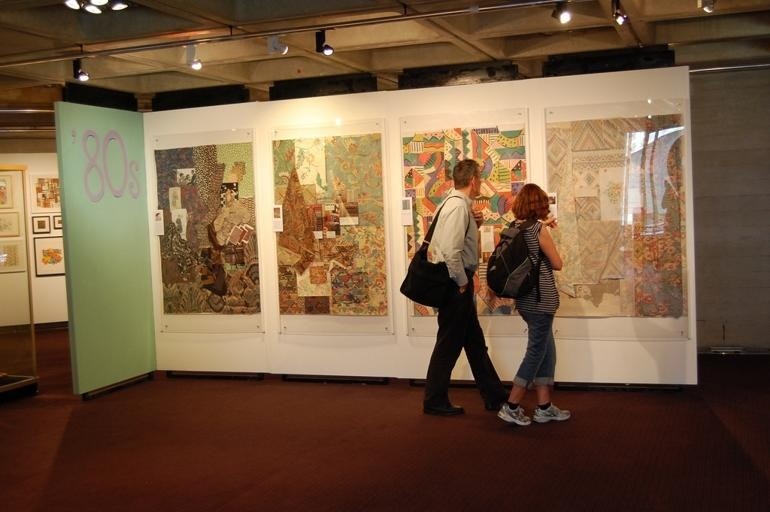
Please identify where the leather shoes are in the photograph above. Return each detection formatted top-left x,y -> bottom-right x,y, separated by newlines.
421,400 -> 464,416
483,387 -> 510,410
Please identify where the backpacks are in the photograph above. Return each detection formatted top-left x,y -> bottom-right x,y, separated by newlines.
485,217 -> 545,299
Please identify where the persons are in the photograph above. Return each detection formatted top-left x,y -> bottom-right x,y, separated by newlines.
423,159 -> 510,416
497,184 -> 571,426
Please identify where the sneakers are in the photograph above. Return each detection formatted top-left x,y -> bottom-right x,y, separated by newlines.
532,402 -> 571,423
496,402 -> 532,426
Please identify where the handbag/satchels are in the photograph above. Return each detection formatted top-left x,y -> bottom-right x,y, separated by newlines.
399,195 -> 471,310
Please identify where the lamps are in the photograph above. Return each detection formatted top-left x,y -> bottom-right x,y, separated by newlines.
315,30 -> 333,56
552,3 -> 571,23
73,58 -> 90,82
697,1 -> 716,13
64,1 -> 129,15
610,0 -> 628,25
186,44 -> 202,69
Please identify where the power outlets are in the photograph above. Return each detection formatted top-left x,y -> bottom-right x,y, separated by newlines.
271,37 -> 288,56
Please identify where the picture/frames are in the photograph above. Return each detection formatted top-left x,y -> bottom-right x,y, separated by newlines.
1,174 -> 25,274
29,173 -> 66,276
